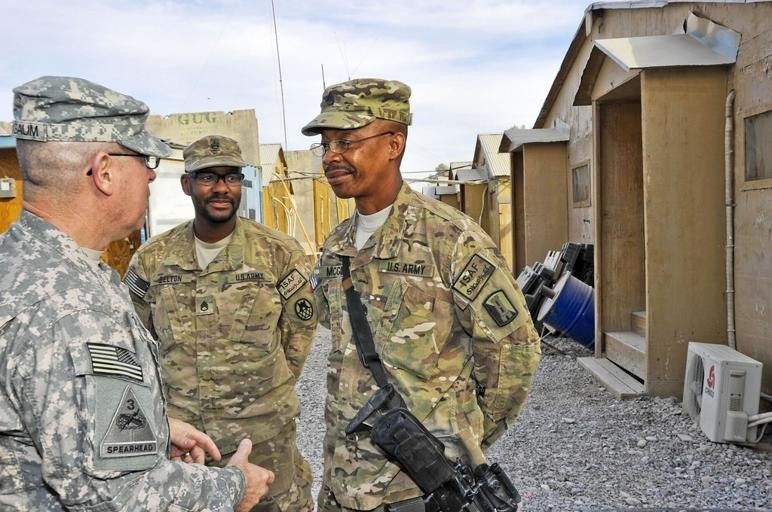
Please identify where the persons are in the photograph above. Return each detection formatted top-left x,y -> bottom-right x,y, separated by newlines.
121,136 -> 321,510
301,80 -> 541,512
0,76 -> 275,511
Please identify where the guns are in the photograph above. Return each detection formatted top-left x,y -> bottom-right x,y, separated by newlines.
340,386 -> 522,512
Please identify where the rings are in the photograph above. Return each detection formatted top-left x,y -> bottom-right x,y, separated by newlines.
181,452 -> 191,460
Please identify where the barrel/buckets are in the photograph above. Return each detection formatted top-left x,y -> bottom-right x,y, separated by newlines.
537,271 -> 595,352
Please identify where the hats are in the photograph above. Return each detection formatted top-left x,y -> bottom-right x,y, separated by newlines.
11,76 -> 174,159
301,78 -> 413,136
182,135 -> 247,173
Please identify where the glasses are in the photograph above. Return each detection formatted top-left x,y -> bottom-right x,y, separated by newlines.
86,153 -> 160,176
308,133 -> 392,157
191,172 -> 244,187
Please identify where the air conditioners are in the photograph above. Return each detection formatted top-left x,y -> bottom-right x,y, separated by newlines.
681,341 -> 772,447
